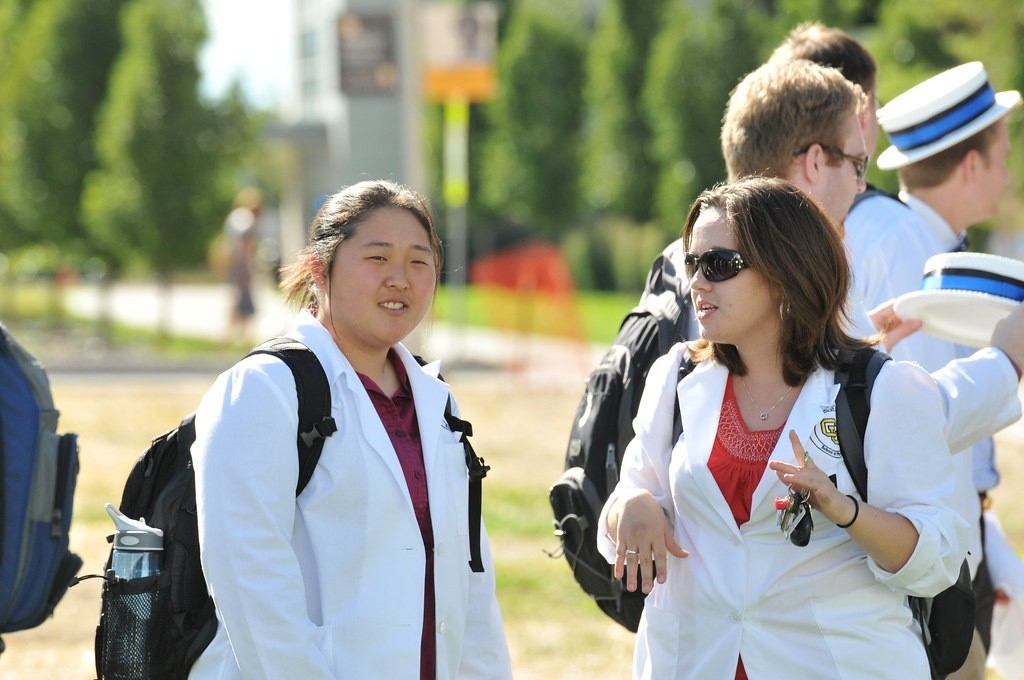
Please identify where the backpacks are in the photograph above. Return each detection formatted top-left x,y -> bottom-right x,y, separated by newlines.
96,411 -> 217,680
0,324 -> 85,640
907,551 -> 976,679
550,241 -> 692,633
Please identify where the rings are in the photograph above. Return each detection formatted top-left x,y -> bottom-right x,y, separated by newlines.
625,548 -> 638,555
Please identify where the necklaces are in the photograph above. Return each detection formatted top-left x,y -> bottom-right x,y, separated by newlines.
741,372 -> 795,420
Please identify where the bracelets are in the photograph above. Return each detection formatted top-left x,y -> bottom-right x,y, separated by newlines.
835,494 -> 859,528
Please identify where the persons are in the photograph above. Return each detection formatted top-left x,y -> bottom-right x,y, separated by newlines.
181,180 -> 515,680
873,61 -> 1024,674
596,175 -> 968,680
771,21 -> 924,341
637,58 -> 1023,456
224,184 -> 262,344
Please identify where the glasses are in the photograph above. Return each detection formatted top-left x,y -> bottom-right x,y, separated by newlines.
789,141 -> 873,180
683,247 -> 750,282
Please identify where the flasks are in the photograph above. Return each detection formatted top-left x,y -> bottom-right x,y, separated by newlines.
103,504 -> 163,680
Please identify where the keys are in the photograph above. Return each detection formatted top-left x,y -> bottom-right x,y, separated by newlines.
774,450 -> 815,548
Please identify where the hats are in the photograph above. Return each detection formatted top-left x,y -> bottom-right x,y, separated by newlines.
893,250 -> 1024,347
870,62 -> 1024,171
